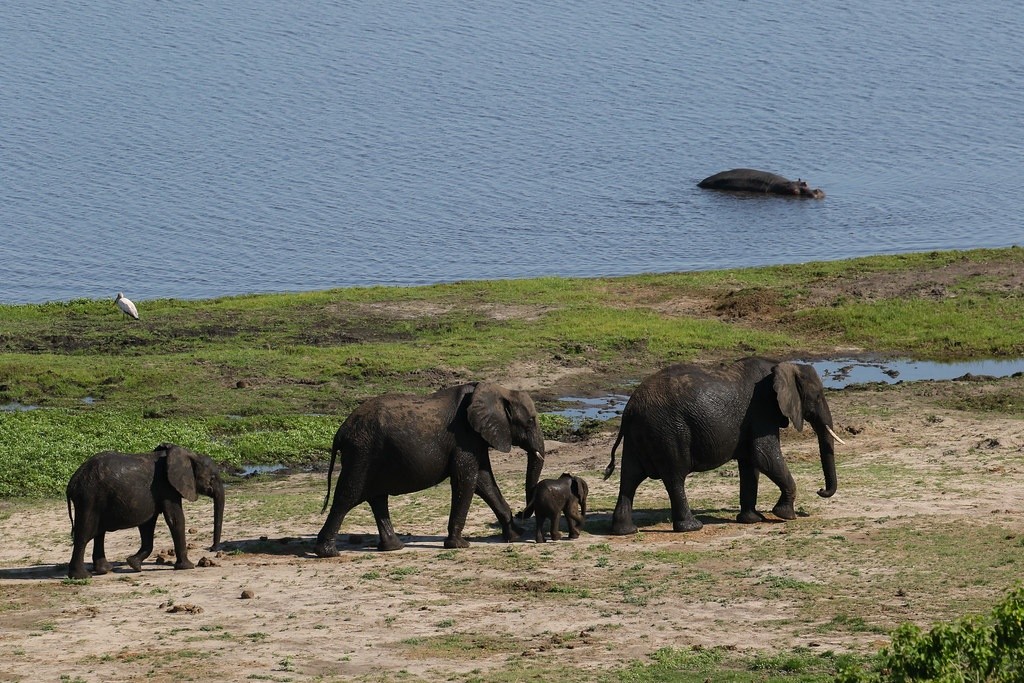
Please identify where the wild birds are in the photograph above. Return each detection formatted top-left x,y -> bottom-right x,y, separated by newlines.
113,292 -> 139,321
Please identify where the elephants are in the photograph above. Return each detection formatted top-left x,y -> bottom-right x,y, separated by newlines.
315,355 -> 846,559
66,441 -> 225,580
